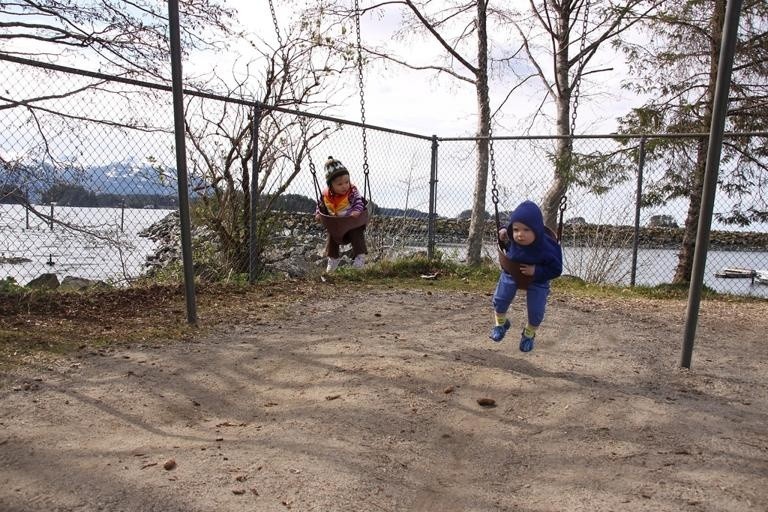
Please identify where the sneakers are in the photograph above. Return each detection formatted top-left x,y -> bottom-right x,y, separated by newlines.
490,319 -> 511,342
352,254 -> 365,269
519,327 -> 535,352
326,257 -> 340,273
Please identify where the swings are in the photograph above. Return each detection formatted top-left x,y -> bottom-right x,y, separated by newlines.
475,0 -> 591,289
269,0 -> 374,244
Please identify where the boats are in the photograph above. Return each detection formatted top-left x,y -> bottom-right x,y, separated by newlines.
712,265 -> 767,285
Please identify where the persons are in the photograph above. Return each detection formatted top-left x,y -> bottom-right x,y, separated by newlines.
491,200 -> 563,352
316,156 -> 368,273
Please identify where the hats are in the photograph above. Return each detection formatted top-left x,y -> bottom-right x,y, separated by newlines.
324,156 -> 349,186
